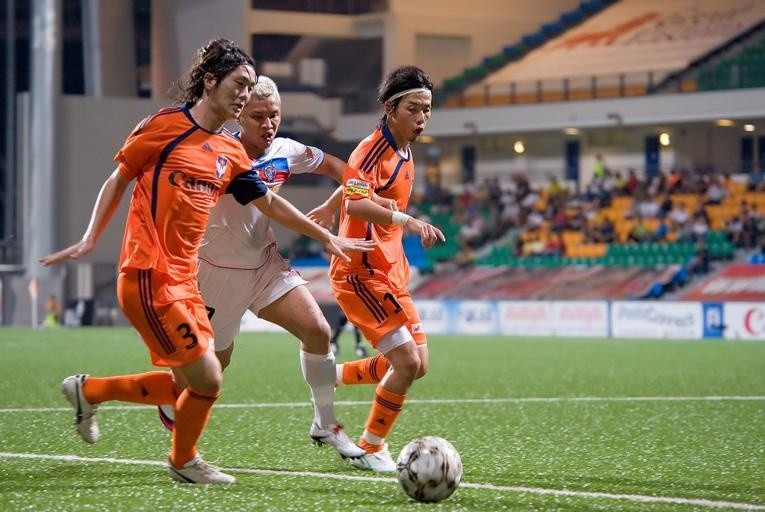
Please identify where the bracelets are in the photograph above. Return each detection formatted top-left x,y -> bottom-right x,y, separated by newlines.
389,210 -> 413,226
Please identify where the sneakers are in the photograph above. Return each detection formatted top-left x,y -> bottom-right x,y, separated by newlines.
167,454 -> 235,483
60,374 -> 101,443
309,421 -> 366,459
352,443 -> 396,473
157,404 -> 176,429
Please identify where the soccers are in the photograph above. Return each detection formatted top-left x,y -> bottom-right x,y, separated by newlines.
397,437 -> 462,503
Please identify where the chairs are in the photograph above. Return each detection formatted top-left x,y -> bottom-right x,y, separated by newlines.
277,202 -> 732,265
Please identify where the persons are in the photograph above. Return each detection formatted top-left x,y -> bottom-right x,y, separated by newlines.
423,152 -> 765,274
159,74 -> 400,461
45,291 -> 61,326
38,39 -> 380,486
304,65 -> 447,473
